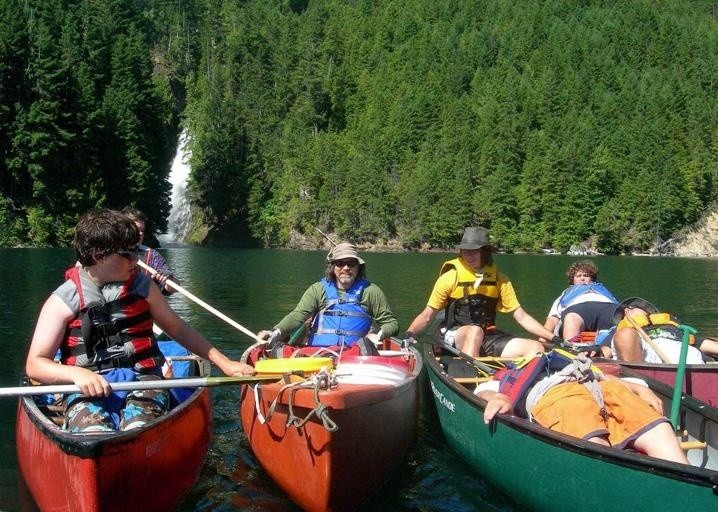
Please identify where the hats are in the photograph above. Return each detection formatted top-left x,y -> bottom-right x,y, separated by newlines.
326,243 -> 366,264
452,227 -> 499,252
612,297 -> 660,325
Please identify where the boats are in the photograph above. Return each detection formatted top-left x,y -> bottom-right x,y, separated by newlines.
15,353 -> 213,512
239,335 -> 423,512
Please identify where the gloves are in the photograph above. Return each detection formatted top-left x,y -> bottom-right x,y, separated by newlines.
257,329 -> 279,345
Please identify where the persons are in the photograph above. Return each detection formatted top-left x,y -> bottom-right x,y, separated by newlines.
121,209 -> 180,341
256,244 -> 400,350
475,349 -> 692,467
577,298 -> 718,364
24,208 -> 257,436
540,261 -> 621,343
399,228 -> 571,371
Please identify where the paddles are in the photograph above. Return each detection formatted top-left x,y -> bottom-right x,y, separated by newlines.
1,375 -> 283,395
420,330 -> 498,374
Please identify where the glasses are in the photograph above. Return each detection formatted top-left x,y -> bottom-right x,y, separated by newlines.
335,260 -> 358,268
101,242 -> 140,261
